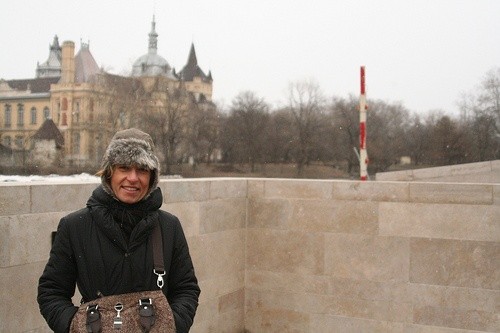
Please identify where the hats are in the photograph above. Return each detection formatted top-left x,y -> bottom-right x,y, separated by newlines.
100,128 -> 159,202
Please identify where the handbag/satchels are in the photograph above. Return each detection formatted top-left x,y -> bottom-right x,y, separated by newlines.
69,290 -> 176,333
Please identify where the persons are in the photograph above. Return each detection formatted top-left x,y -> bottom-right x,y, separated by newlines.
37,128 -> 201,333
